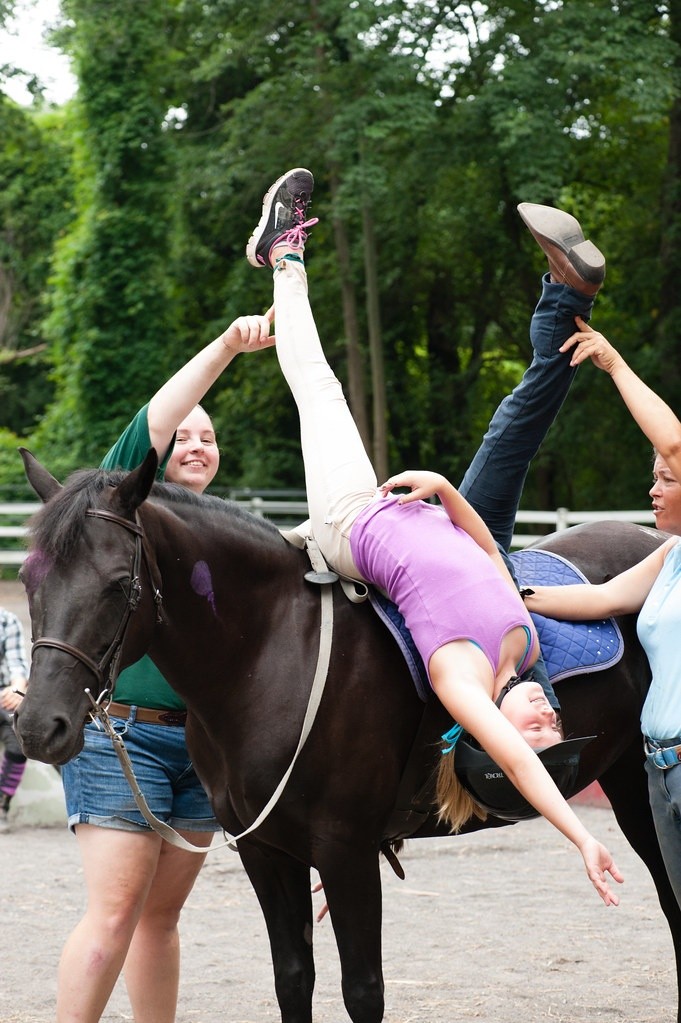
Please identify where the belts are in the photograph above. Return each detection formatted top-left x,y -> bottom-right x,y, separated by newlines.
99,700 -> 187,728
644,742 -> 681,770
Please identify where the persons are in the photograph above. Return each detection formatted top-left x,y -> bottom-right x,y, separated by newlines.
0,606 -> 32,833
516,316 -> 681,913
52,299 -> 278,1023
455,201 -> 609,736
244,167 -> 624,908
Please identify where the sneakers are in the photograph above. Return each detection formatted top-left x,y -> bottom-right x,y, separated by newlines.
245,168 -> 315,267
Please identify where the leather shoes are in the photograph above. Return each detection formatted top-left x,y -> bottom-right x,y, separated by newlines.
516,202 -> 606,297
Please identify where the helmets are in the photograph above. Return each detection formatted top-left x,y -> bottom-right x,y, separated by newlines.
454,735 -> 616,822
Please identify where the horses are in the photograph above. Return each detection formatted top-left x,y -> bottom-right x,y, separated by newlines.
13,445 -> 680,1023
